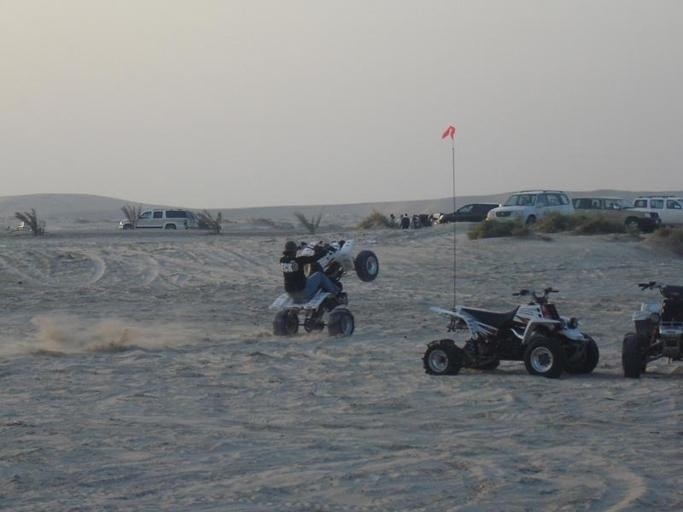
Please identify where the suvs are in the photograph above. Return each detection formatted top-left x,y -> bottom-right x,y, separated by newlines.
117,207 -> 193,232
630,195 -> 683,227
571,196 -> 663,234
438,203 -> 501,226
485,189 -> 577,228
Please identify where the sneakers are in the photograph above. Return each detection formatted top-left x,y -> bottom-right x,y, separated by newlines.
335,282 -> 342,297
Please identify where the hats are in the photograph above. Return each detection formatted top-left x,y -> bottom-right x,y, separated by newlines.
283,241 -> 297,254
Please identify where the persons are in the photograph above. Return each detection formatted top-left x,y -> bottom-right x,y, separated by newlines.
388,213 -> 443,230
279,241 -> 345,304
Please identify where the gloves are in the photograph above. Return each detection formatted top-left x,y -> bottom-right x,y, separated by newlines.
316,247 -> 327,256
